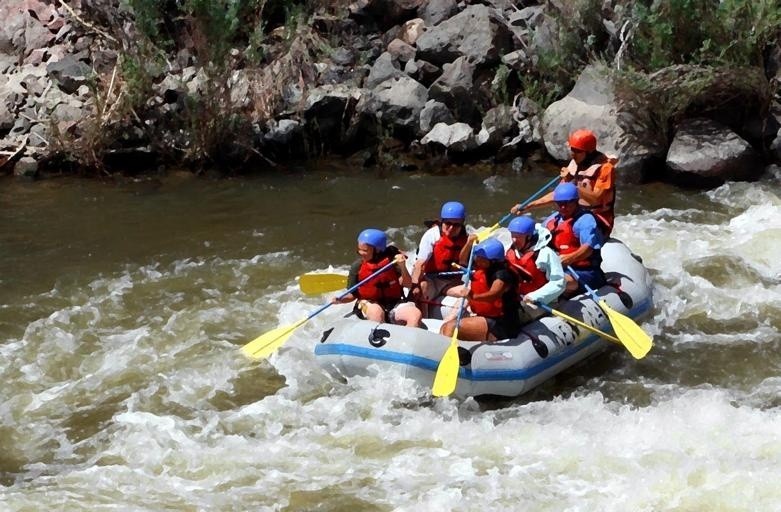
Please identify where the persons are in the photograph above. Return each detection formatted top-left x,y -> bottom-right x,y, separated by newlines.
540,182 -> 602,301
329,228 -> 423,328
510,128 -> 616,245
439,237 -> 521,343
408,201 -> 469,318
504,216 -> 566,327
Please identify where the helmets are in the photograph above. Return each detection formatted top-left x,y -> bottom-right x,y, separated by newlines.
508,216 -> 534,234
473,239 -> 504,260
554,183 -> 578,201
569,130 -> 596,152
358,229 -> 386,253
441,201 -> 464,219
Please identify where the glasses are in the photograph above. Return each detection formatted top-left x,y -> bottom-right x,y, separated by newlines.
571,147 -> 582,154
442,220 -> 462,227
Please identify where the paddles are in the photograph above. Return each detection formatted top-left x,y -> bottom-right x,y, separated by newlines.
432,234 -> 476,397
238,254 -> 398,359
452,263 -> 621,345
299,273 -> 476,295
554,250 -> 652,359
473,175 -> 561,243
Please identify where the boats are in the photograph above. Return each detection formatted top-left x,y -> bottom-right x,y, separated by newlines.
310,237 -> 653,402
